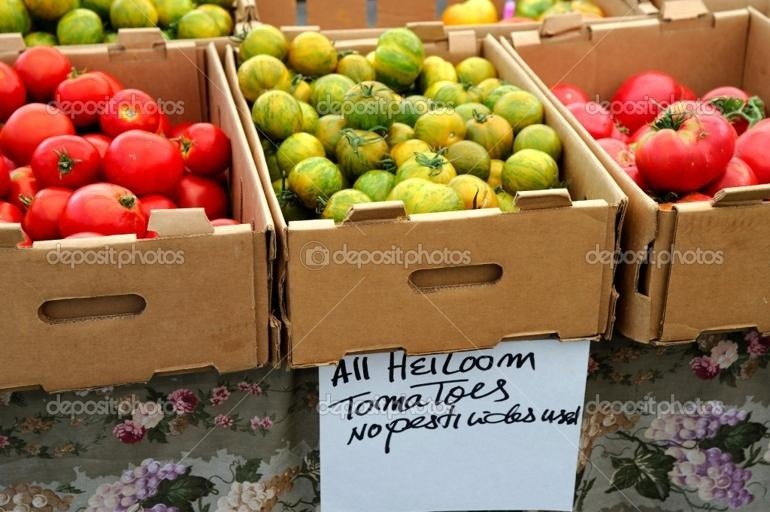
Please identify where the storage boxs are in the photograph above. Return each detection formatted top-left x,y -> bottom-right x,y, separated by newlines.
503,2 -> 770,347
0,29 -> 277,399
227,30 -> 634,373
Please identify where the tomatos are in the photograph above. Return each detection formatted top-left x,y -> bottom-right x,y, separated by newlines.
0,0 -> 770,247
